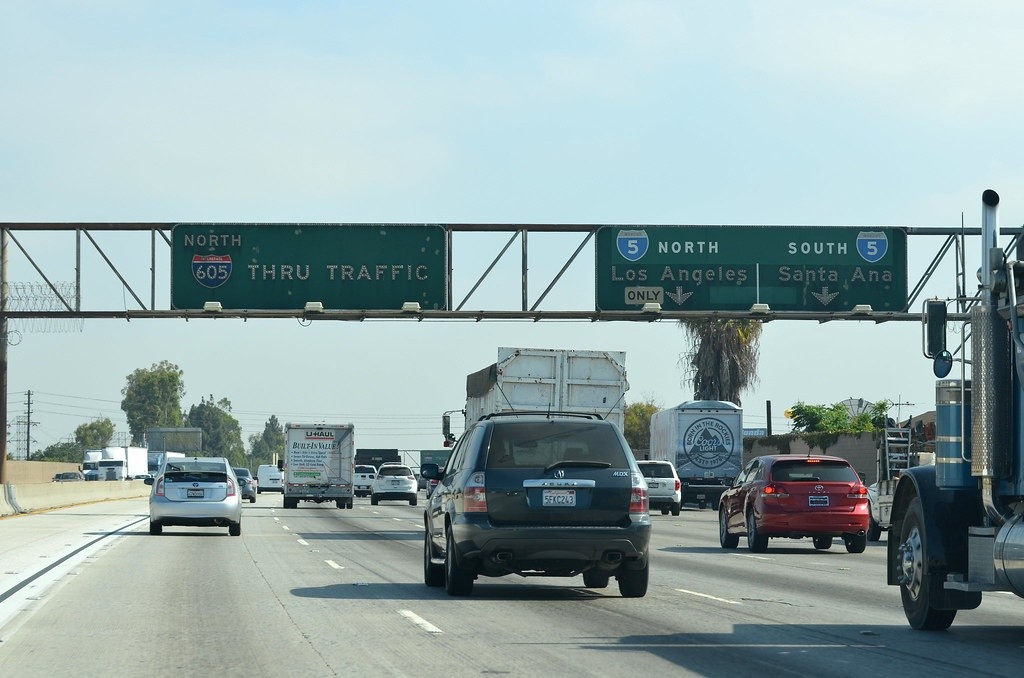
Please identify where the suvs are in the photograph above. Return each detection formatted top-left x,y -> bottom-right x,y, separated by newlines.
636,459 -> 682,516
420,410 -> 652,597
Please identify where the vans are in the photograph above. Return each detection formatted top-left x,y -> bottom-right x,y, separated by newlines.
256,464 -> 284,496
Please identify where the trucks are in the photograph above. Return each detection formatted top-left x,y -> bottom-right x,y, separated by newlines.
277,422 -> 355,509
645,401 -> 744,511
887,189 -> 1024,631
868,427 -> 921,541
443,346 -> 630,446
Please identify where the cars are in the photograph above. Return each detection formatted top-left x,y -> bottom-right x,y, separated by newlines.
352,464 -> 377,497
369,464 -> 418,506
52,473 -> 86,482
719,454 -> 870,554
233,466 -> 259,503
144,456 -> 246,536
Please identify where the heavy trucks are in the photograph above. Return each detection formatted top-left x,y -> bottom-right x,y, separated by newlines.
97,447 -> 148,480
147,451 -> 185,478
78,450 -> 102,481
